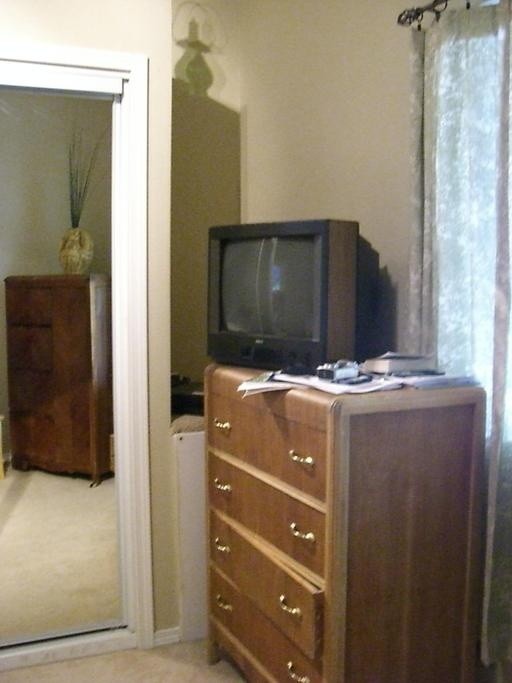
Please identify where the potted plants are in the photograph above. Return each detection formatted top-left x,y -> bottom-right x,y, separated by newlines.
60,120 -> 111,274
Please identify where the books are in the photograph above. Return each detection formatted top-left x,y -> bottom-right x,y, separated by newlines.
363,351 -> 436,375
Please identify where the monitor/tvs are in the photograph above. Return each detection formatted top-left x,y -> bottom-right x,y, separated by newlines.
205,218 -> 391,377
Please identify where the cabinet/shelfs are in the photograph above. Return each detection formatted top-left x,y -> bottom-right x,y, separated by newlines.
203,368 -> 486,683
7,274 -> 113,485
